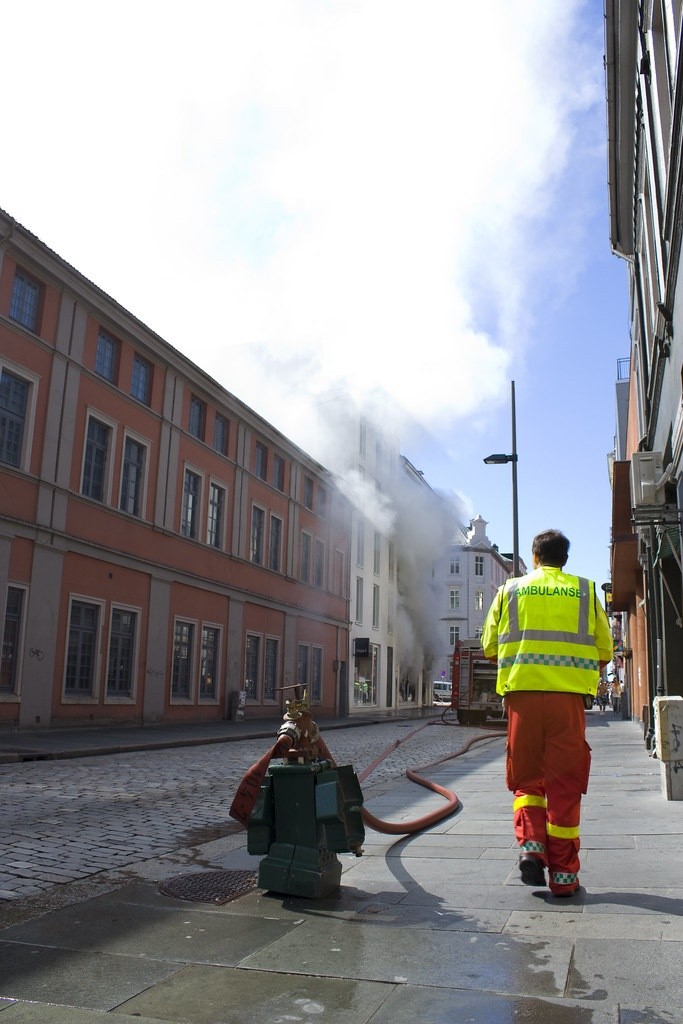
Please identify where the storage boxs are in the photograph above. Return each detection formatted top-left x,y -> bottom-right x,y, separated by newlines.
660,760 -> 683,802
652,695 -> 683,762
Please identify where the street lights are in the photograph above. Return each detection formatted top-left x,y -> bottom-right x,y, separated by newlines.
482,381 -> 519,577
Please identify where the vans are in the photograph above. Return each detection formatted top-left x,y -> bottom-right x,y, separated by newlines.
432,683 -> 453,701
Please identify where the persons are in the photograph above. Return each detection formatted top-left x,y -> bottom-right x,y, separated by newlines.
481,530 -> 624,895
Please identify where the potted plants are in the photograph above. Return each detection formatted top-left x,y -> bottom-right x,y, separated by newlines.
621,646 -> 632,659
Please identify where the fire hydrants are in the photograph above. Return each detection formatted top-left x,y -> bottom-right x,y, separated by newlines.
247,692 -> 365,898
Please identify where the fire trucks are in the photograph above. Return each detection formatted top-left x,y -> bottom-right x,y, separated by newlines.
452,638 -> 503,726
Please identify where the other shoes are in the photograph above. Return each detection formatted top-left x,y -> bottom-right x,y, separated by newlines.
600,711 -> 604,716
518,855 -> 547,887
